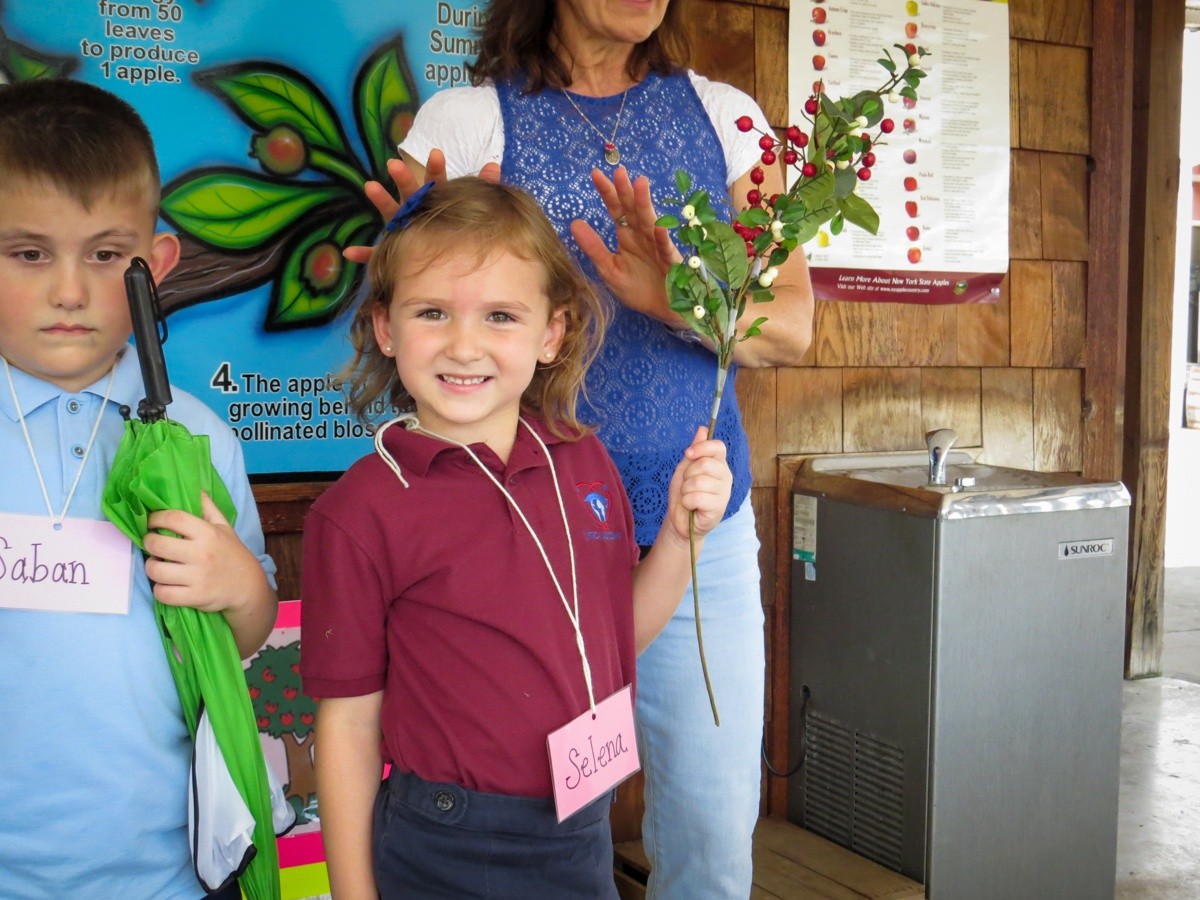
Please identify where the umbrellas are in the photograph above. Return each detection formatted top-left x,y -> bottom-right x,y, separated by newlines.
105,253 -> 279,900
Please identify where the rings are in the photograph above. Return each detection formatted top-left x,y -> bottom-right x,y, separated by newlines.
615,214 -> 629,227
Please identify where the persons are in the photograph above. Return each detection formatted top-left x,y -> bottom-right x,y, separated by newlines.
300,176 -> 735,900
0,77 -> 278,900
343,0 -> 815,900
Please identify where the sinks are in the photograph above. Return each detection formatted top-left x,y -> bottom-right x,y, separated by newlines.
843,464 -> 1059,495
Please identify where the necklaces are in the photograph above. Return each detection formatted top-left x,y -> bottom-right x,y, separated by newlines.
549,68 -> 631,165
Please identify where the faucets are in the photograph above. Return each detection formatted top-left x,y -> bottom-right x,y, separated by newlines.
924,427 -> 958,486
